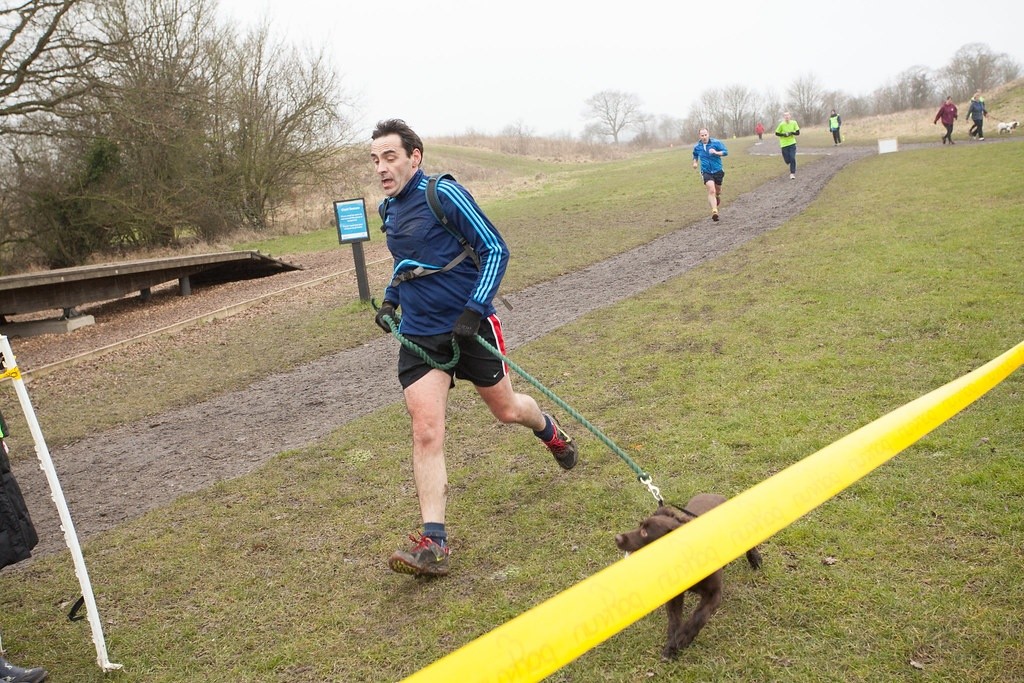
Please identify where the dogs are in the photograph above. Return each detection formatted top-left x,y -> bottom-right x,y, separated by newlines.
998,121 -> 1019,135
614,493 -> 763,660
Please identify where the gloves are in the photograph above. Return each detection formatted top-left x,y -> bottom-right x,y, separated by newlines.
451,310 -> 480,341
375,302 -> 398,333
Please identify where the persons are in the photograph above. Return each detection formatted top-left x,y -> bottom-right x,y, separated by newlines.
966,89 -> 988,141
693,129 -> 728,222
756,123 -> 764,139
0,407 -> 48,683
934,97 -> 958,144
829,110 -> 842,144
776,112 -> 801,179
374,119 -> 578,578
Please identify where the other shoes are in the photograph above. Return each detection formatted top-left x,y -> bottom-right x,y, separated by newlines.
715,192 -> 721,205
790,173 -> 795,180
943,137 -> 946,144
971,134 -> 976,140
979,137 -> 985,141
0,657 -> 47,683
712,211 -> 719,221
949,139 -> 955,144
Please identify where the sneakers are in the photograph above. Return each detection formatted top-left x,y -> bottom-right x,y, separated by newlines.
539,413 -> 578,470
388,530 -> 450,575
